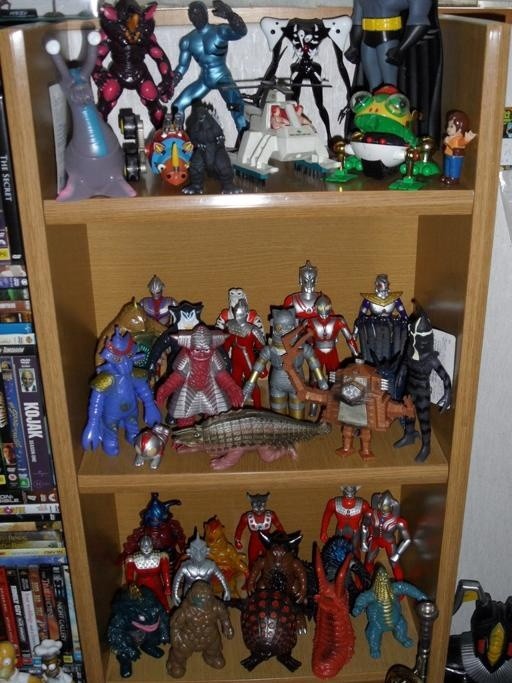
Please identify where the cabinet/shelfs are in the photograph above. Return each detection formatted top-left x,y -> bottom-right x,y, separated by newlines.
0,7 -> 511,683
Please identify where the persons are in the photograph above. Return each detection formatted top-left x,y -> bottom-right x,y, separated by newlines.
33,639 -> 76,683
0,641 -> 31,683
20,371 -> 36,392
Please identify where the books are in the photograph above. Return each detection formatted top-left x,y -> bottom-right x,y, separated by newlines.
0,513 -> 83,683
1,88 -> 37,346
3,346 -> 61,515
